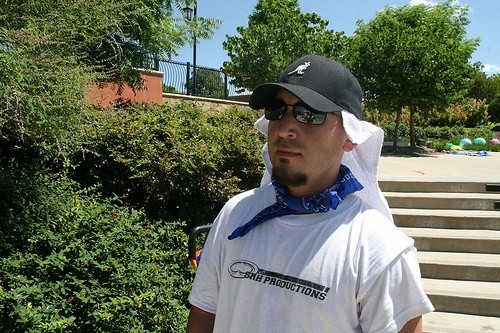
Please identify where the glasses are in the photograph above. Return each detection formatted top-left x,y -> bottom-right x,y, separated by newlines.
264,98 -> 342,126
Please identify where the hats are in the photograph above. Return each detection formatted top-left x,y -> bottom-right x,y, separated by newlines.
247,55 -> 363,122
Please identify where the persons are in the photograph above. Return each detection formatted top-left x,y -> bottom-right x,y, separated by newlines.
186,54 -> 436,333
